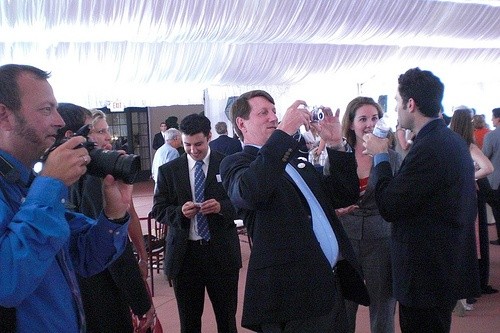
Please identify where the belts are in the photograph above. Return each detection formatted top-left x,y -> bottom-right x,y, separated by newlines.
189,240 -> 209,245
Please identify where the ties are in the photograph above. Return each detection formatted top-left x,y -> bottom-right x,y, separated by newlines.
193,160 -> 211,242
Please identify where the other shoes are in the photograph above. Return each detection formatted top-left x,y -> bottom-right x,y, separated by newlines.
485,285 -> 499,295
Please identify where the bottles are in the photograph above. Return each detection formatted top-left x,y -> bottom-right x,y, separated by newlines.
372,112 -> 392,139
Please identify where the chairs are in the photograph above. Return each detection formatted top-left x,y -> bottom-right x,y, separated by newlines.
131,210 -> 167,289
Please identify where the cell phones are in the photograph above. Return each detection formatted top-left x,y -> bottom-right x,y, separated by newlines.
193,203 -> 202,207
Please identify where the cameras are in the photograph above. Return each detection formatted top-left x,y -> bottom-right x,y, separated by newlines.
53,124 -> 141,184
303,105 -> 324,122
